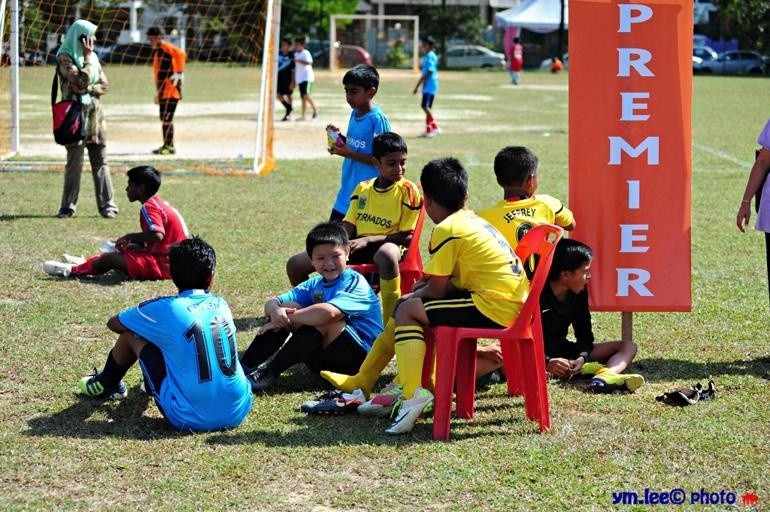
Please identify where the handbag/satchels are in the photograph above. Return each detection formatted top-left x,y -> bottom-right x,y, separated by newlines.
154,90 -> 160,104
53,100 -> 85,145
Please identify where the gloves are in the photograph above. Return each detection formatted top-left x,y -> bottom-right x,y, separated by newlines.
169,72 -> 184,87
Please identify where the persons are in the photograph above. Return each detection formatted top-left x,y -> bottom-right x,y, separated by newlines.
56,20 -> 119,218
326,64 -> 392,223
507,37 -> 525,84
733,110 -> 769,305
235,131 -> 650,435
145,27 -> 188,155
412,36 -> 443,137
1,32 -> 63,67
43,165 -> 192,281
291,37 -> 318,121
277,37 -> 296,121
548,54 -> 564,73
78,234 -> 254,432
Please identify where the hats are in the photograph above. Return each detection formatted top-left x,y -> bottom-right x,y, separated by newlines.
147,27 -> 165,35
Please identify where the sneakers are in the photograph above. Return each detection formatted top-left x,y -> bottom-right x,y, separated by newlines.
423,126 -> 441,138
587,367 -> 644,394
100,211 -> 115,218
282,108 -> 319,122
152,144 -> 175,154
580,361 -> 603,375
57,209 -> 71,218
140,372 -> 146,393
79,367 -> 128,402
301,383 -> 434,433
43,253 -> 86,277
240,362 -> 280,393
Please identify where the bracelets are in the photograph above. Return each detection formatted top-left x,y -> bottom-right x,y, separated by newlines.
741,197 -> 752,205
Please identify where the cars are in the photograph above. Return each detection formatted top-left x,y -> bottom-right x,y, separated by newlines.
311,45 -> 372,68
693,47 -> 717,60
701,50 -> 766,76
403,46 -> 508,72
693,55 -> 702,73
539,53 -> 569,71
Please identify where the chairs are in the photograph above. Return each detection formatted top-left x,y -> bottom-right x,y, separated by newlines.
348,182 -> 424,294
423,223 -> 565,440
